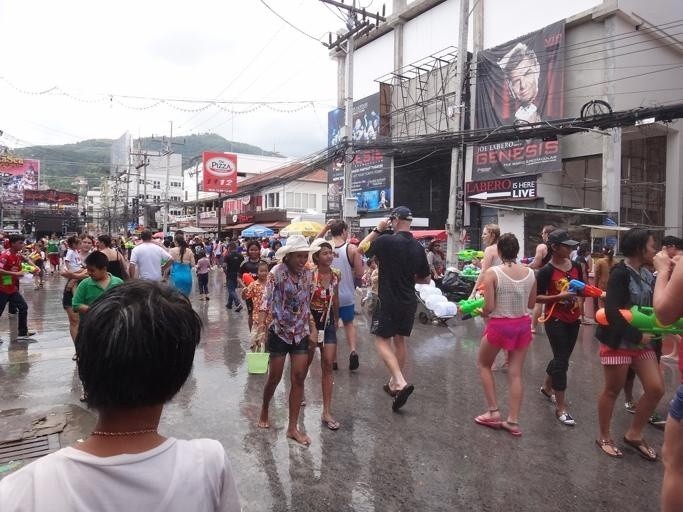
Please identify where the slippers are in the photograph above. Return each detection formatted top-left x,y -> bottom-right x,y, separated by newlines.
624,434 -> 658,461
323,415 -> 339,430
384,383 -> 414,410
555,408 -> 575,426
596,437 -> 623,458
539,386 -> 558,404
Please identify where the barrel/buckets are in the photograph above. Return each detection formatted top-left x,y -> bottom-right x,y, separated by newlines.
414,279 -> 457,317
246,343 -> 271,374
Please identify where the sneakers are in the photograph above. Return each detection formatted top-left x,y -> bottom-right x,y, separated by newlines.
624,400 -> 637,413
19,332 -> 36,337
649,410 -> 666,425
226,304 -> 242,312
349,351 -> 359,370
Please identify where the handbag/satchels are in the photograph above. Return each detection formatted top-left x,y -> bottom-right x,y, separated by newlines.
316,330 -> 325,347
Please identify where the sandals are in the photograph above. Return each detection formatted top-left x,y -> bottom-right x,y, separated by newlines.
474,409 -> 522,436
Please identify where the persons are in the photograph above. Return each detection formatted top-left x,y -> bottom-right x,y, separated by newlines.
377,190 -> 390,210
496,42 -> 542,129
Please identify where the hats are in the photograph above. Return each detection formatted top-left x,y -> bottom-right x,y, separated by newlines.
550,229 -> 580,246
309,238 -> 335,265
275,235 -> 320,260
386,206 -> 413,221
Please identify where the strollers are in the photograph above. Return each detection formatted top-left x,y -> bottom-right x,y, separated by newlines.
435,266 -> 474,301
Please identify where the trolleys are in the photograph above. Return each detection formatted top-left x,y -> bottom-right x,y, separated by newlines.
415,288 -> 456,327
